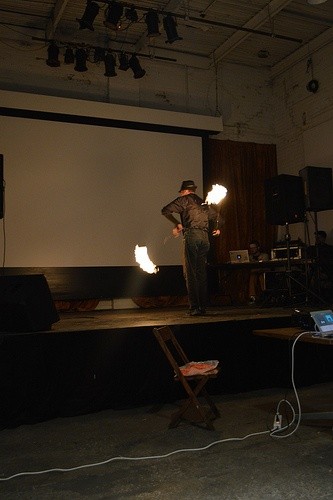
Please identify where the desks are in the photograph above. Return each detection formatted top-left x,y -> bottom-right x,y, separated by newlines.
254,326 -> 333,422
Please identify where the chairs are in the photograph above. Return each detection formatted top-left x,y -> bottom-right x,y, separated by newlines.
153,324 -> 225,431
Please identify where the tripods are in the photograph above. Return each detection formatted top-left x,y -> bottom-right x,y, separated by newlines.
257,223 -> 331,309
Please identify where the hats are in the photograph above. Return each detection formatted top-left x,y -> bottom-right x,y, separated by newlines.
314,231 -> 327,237
178,181 -> 196,193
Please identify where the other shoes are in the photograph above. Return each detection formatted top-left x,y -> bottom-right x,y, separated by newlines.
199,306 -> 206,315
248,296 -> 256,305
185,306 -> 201,316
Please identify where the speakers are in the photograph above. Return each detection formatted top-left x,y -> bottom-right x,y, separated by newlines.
264,174 -> 306,226
0,274 -> 58,332
305,166 -> 333,212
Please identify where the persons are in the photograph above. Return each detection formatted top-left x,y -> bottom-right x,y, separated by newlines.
314,231 -> 327,248
161,180 -> 224,317
248,242 -> 269,304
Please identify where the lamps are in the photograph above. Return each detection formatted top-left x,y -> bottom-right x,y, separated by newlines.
75,1 -> 100,31
103,3 -> 124,30
74,47 -> 88,72
118,53 -> 130,72
94,48 -> 105,64
127,7 -> 138,20
47,42 -> 61,67
105,51 -> 117,77
129,54 -> 145,79
163,14 -> 183,44
65,45 -> 75,64
145,8 -> 161,38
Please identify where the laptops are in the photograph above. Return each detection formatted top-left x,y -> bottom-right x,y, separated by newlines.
229,250 -> 251,264
310,310 -> 333,337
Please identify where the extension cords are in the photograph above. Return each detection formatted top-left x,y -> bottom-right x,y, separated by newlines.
274,414 -> 283,429
